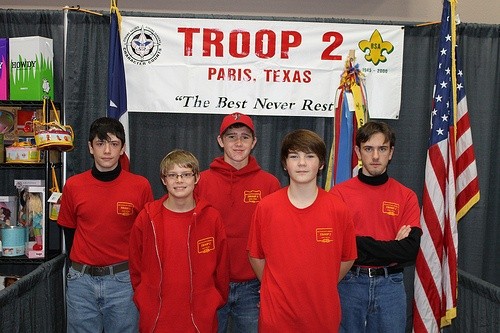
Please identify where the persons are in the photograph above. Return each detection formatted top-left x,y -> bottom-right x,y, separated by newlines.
328,119 -> 423,333
247,129 -> 358,333
129,150 -> 230,333
57,117 -> 154,333
194,112 -> 282,333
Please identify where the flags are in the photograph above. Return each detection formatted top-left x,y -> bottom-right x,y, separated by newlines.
412,0 -> 481,333
108,0 -> 130,162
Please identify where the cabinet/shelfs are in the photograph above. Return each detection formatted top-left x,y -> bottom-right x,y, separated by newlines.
0,101 -> 61,266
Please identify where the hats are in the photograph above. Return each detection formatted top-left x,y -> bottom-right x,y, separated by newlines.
220,113 -> 255,136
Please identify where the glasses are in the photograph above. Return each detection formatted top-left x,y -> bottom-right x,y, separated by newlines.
164,173 -> 196,177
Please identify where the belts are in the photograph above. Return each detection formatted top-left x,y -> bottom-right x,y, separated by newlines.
71,261 -> 127,277
352,263 -> 404,277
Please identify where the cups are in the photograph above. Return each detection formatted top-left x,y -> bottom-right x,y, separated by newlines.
0,226 -> 29,257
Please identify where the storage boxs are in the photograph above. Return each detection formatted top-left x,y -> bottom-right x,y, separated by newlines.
0,38 -> 9,100
0,196 -> 17,253
25,186 -> 45,259
14,180 -> 45,227
9,36 -> 54,101
